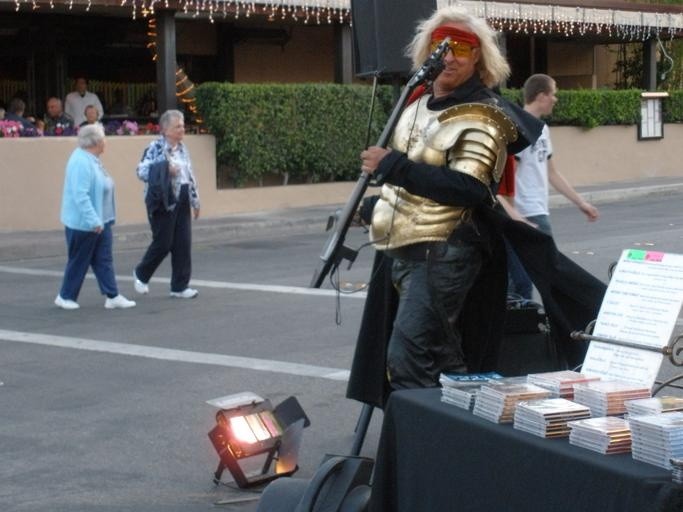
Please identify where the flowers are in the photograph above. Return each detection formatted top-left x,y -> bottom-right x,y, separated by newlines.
1,120 -> 160,136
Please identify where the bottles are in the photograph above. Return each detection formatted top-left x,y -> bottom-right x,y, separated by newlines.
54,123 -> 63,136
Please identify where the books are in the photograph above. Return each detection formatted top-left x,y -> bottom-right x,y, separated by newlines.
624,396 -> 683,424
436,372 -> 511,411
473,382 -> 551,424
512,398 -> 592,438
669,457 -> 682,484
573,378 -> 651,416
626,411 -> 683,471
526,370 -> 600,402
567,415 -> 634,456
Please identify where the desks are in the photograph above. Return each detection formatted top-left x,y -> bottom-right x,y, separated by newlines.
101,114 -> 156,126
365,373 -> 683,511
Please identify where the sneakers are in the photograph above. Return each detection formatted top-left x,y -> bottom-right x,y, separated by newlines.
55,293 -> 80,310
133,269 -> 150,296
170,287 -> 200,300
105,294 -> 135,310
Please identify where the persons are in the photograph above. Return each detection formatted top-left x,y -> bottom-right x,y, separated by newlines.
340,9 -> 510,409
496,74 -> 597,300
52,122 -> 136,309
133,110 -> 201,299
0,76 -> 105,137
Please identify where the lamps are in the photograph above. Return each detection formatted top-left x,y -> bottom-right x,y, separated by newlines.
209,397 -> 310,486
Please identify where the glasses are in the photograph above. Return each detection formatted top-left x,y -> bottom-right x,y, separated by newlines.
431,40 -> 475,59
